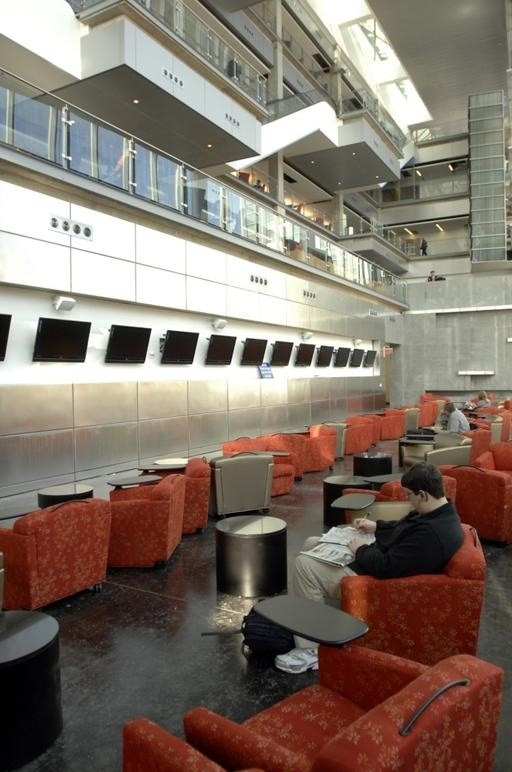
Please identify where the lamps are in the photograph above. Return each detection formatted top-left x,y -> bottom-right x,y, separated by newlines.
303,332 -> 312,339
354,338 -> 362,345
213,318 -> 228,329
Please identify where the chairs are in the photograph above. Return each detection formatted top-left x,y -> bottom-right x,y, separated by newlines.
123,718 -> 262,772
185,596 -> 504,772
341,524 -> 487,666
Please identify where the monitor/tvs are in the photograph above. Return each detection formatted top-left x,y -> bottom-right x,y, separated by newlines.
295,343 -> 315,367
364,350 -> 377,368
239,337 -> 268,366
30,316 -> 91,364
203,334 -> 237,366
270,340 -> 294,366
317,345 -> 334,367
350,348 -> 364,367
104,324 -> 152,366
334,347 -> 351,367
0,313 -> 12,363
159,330 -> 200,365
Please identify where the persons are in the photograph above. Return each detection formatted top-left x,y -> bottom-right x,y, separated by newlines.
475,391 -> 490,408
275,460 -> 464,674
435,403 -> 471,434
420,238 -> 428,255
427,271 -> 438,281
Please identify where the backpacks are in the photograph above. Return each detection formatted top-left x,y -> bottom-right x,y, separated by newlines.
242,599 -> 296,652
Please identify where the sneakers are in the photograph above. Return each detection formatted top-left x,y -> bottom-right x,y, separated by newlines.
276,647 -> 319,672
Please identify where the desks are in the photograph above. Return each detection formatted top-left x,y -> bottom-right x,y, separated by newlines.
0,610 -> 63,772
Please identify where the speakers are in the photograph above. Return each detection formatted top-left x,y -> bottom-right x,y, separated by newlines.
352,338 -> 362,347
300,331 -> 314,340
211,317 -> 229,329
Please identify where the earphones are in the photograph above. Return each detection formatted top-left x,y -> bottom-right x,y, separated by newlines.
419,490 -> 425,499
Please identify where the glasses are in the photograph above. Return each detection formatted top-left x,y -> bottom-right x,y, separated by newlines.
406,490 -> 423,501
